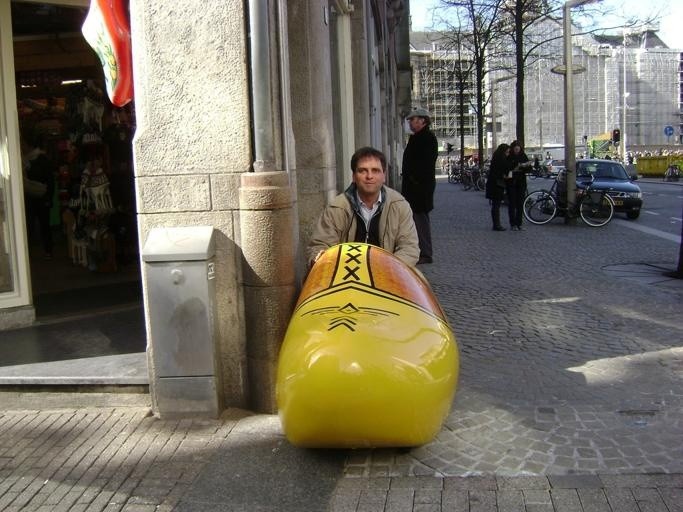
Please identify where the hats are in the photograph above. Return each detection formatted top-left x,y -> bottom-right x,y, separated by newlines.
406,108 -> 431,120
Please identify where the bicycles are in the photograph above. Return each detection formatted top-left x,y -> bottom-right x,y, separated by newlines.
443,155 -> 491,192
527,173 -> 614,222
522,168 -> 615,228
528,164 -> 550,180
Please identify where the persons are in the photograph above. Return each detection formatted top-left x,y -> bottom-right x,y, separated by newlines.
400,109 -> 438,263
306,146 -> 419,268
483,143 -> 516,231
20,135 -> 57,260
508,140 -> 534,232
532,147 -> 666,179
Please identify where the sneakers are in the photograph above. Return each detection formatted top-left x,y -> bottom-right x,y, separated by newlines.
518,226 -> 527,231
492,225 -> 506,231
511,225 -> 519,231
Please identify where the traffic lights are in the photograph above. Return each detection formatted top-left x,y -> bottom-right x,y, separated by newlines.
447,142 -> 454,153
612,129 -> 620,141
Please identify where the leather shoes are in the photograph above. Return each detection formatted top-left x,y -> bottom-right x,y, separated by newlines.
416,257 -> 433,264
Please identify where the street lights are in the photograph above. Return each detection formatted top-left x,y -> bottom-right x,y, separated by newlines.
489,74 -> 515,154
481,86 -> 499,94
562,0 -> 605,226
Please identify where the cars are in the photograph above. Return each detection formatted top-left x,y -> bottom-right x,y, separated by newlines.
525,152 -> 546,170
541,159 -> 565,178
555,159 -> 643,219
